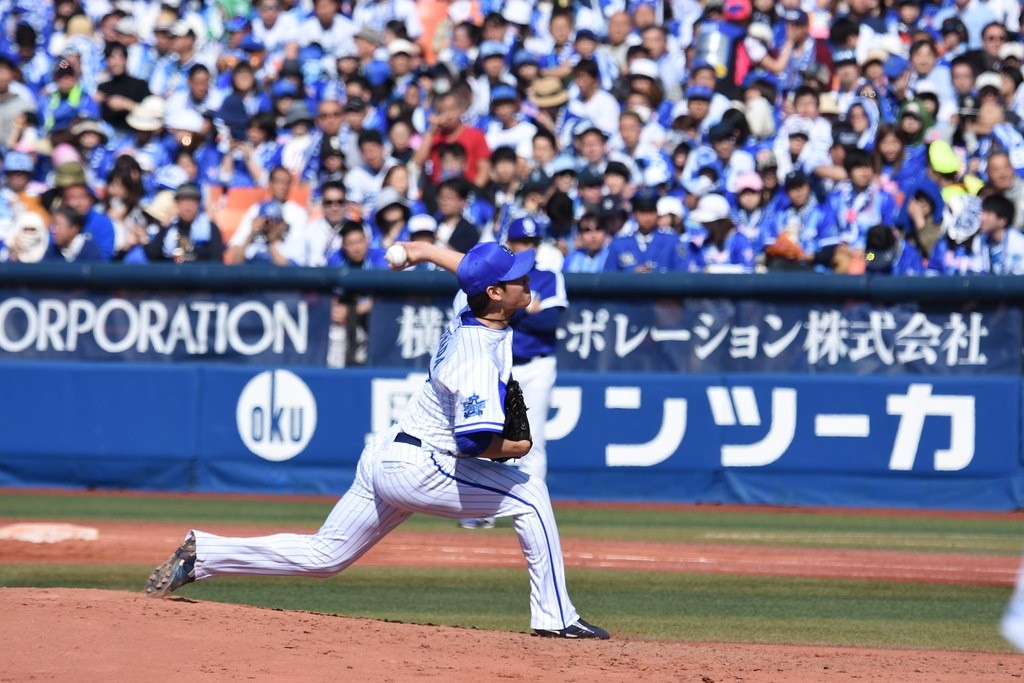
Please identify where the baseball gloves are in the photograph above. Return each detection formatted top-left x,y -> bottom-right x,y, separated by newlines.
490,378 -> 533,464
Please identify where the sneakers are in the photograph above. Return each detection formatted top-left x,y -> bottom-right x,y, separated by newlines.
146,534 -> 196,596
533,617 -> 611,639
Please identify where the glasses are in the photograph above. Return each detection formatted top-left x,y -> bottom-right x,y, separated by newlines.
578,225 -> 605,234
322,198 -> 344,207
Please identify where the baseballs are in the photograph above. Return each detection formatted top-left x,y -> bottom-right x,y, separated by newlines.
386,245 -> 408,267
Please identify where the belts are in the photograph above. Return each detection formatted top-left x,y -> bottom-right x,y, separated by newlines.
394,432 -> 421,447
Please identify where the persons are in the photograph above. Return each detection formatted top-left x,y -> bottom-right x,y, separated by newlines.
453,214 -> 569,529
217,62 -> 275,139
144,241 -> 610,639
0,0 -> 1024,275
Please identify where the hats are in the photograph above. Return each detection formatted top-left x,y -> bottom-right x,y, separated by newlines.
260,203 -> 283,222
509,217 -> 542,239
456,241 -> 536,295
407,214 -> 436,234
5,0 -> 1024,217
